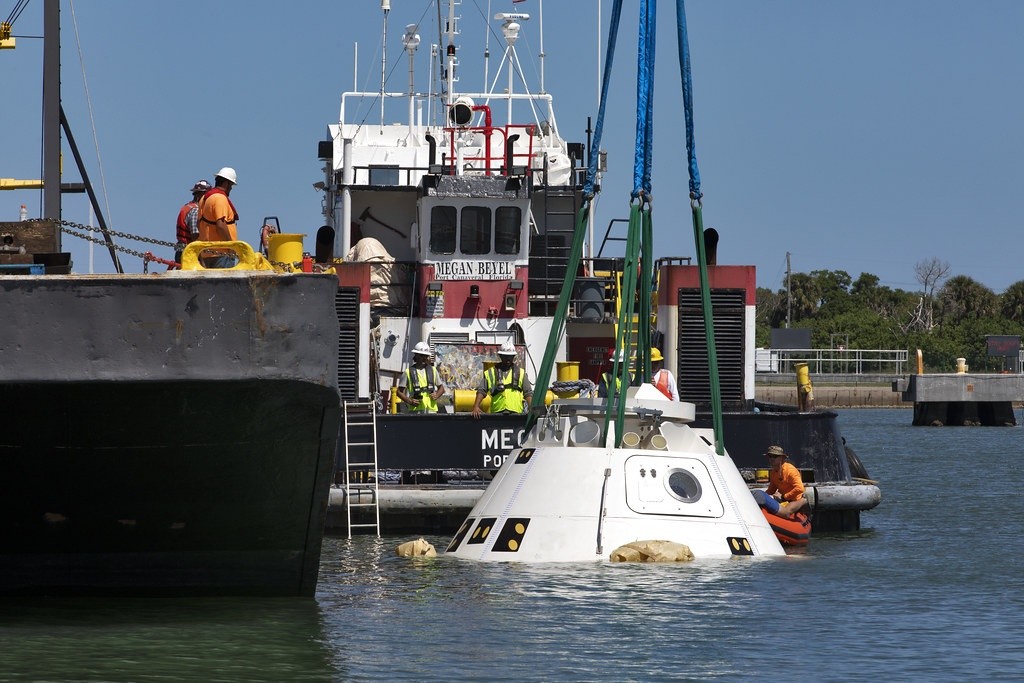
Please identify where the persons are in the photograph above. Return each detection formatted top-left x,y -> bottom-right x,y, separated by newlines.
595,348 -> 640,399
471,341 -> 534,424
744,481 -> 809,523
175,178 -> 212,271
396,340 -> 446,416
197,167 -> 241,270
640,345 -> 680,403
765,445 -> 806,518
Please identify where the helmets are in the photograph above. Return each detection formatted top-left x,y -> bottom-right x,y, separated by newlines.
651,348 -> 664,361
609,349 -> 624,362
497,342 -> 517,355
190,180 -> 211,192
213,167 -> 238,185
411,342 -> 432,356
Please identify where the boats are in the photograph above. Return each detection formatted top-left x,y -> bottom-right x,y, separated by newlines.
0,0 -> 886,600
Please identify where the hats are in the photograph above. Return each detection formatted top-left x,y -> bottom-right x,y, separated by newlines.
761,446 -> 786,456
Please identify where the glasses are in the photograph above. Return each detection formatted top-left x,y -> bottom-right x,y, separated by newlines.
768,454 -> 779,458
